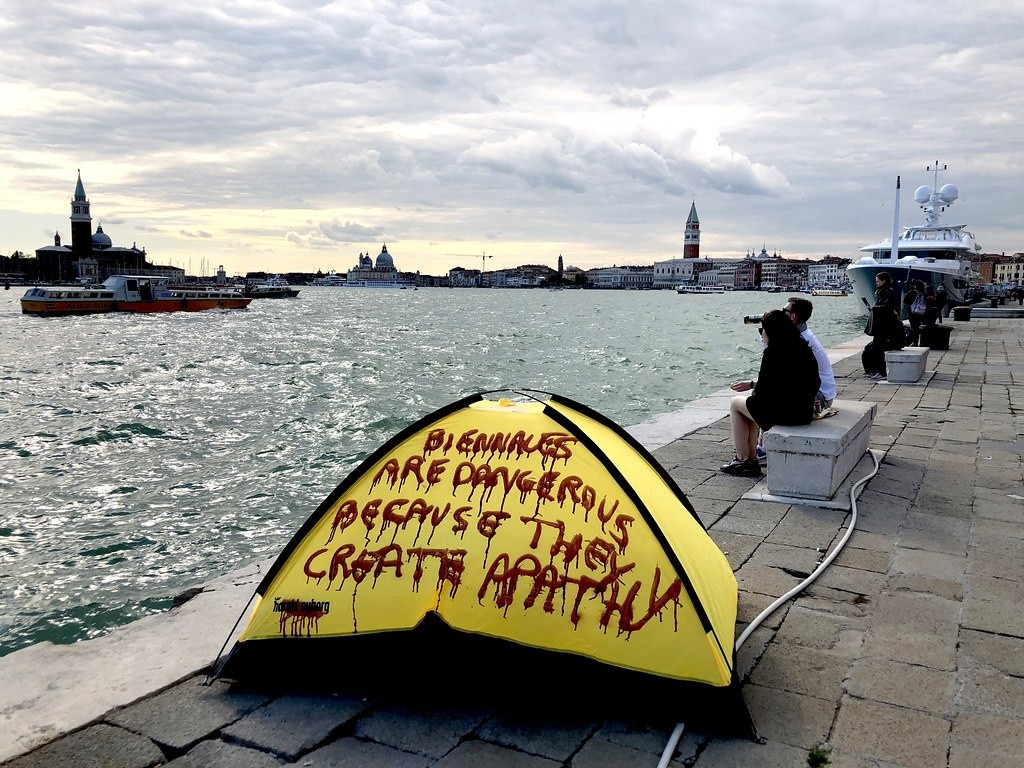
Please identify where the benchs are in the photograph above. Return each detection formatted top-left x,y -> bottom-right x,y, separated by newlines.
884,346 -> 930,383
762,399 -> 878,503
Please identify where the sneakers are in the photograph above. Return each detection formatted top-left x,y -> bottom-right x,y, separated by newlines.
756,445 -> 767,465
720,457 -> 761,476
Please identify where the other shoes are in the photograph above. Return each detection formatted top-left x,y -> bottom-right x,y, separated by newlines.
863,372 -> 887,380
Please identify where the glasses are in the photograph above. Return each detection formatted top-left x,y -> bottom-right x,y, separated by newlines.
782,308 -> 793,314
758,327 -> 764,335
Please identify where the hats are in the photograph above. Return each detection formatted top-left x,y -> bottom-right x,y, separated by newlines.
937,285 -> 944,290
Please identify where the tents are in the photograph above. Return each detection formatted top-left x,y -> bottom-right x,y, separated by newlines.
207,385 -> 767,750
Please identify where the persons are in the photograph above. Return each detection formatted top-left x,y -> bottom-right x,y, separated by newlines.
898,277 -> 951,347
718,309 -> 823,480
777,296 -> 838,421
861,261 -> 896,382
860,307 -> 906,377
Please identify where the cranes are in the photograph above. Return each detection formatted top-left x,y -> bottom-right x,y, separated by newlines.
444,251 -> 493,272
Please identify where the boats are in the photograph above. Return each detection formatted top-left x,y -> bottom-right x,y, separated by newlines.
237,279 -> 300,298
20,275 -> 250,319
846,159 -> 985,321
676,283 -> 725,294
767,278 -> 854,297
306,271 -> 418,292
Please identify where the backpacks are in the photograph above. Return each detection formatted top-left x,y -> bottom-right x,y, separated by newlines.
910,290 -> 926,315
